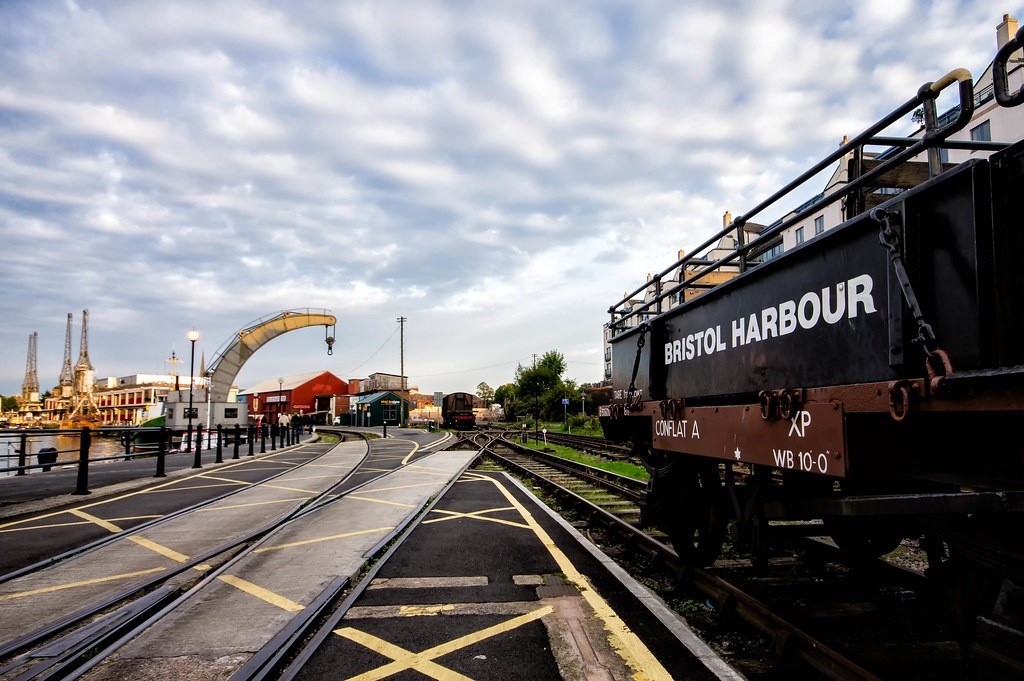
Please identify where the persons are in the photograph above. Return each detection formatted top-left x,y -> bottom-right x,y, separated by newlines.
307,414 -> 314,435
292,413 -> 299,434
279,412 -> 291,437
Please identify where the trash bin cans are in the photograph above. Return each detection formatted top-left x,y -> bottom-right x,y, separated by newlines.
340,413 -> 351,425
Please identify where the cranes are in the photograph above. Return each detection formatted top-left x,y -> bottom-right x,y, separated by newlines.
59,313 -> 72,398
73,310 -> 95,394
19,329 -> 44,415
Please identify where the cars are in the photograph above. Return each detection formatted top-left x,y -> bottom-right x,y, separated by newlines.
332,416 -> 340,426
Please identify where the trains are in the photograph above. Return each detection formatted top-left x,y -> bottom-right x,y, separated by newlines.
439,392 -> 477,430
604,60 -> 1024,673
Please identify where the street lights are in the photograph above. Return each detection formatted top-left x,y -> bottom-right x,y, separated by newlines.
254,393 -> 258,420
278,377 -> 284,415
187,330 -> 200,453
